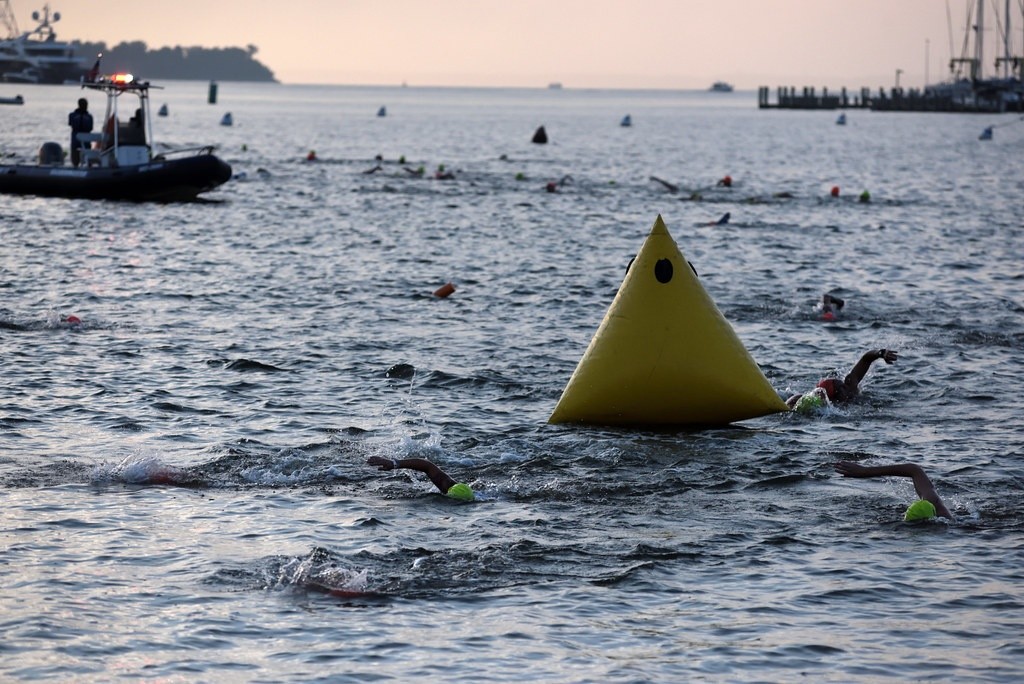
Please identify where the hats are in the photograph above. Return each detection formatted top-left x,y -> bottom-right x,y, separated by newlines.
447,483 -> 475,501
820,313 -> 833,321
817,379 -> 834,400
797,396 -> 824,415
904,500 -> 937,521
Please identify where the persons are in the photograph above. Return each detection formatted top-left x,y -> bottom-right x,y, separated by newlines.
822,294 -> 844,320
0,316 -> 79,330
719,175 -> 732,187
691,192 -> 701,200
367,456 -> 473,499
859,191 -> 870,203
69,99 -> 93,168
831,187 -> 840,196
784,348 -> 898,408
649,176 -> 677,191
834,460 -> 951,521
241,145 -> 616,193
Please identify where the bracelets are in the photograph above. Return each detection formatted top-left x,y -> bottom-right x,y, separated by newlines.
390,458 -> 399,468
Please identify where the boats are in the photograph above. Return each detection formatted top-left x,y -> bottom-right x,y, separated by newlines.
0,5 -> 88,81
710,82 -> 734,92
0,73 -> 232,199
